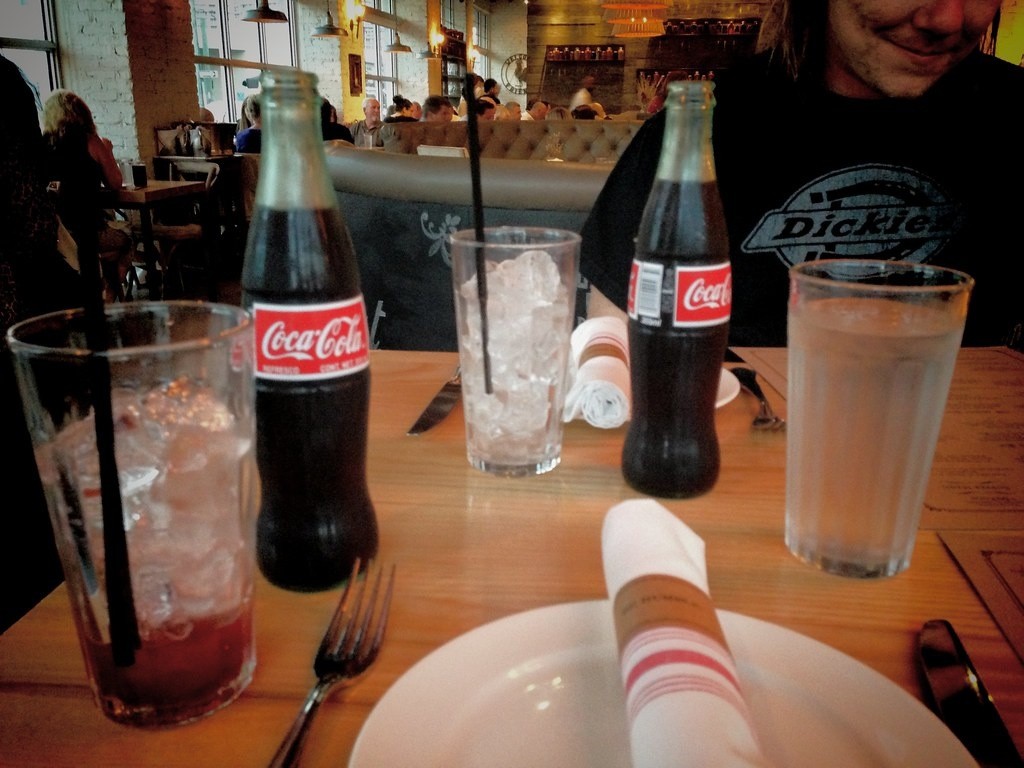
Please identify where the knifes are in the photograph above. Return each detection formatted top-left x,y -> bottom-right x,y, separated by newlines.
913,619 -> 1024,768
405,365 -> 462,437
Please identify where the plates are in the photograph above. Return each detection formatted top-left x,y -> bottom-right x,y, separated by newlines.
714,367 -> 740,408
347,597 -> 978,768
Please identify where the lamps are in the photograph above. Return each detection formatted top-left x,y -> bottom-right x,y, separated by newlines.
382,0 -> 437,60
240,0 -> 287,24
310,0 -> 348,37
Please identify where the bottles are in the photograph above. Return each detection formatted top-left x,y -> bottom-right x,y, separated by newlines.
619,80 -> 735,498
238,68 -> 380,592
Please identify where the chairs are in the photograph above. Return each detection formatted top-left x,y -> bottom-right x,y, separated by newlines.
112,161 -> 220,302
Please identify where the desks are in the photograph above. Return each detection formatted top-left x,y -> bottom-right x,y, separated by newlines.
100,180 -> 205,299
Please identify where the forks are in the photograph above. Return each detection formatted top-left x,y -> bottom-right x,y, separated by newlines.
722,347 -> 785,433
266,558 -> 396,767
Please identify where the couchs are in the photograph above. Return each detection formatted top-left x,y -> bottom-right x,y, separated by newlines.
381,119 -> 648,163
318,138 -> 615,352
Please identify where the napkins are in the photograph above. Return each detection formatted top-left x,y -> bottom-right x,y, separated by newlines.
562,313 -> 635,427
601,496 -> 755,766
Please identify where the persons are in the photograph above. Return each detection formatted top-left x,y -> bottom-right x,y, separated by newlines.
574,0 -> 1024,349
0,52 -> 134,628
197,72 -> 686,153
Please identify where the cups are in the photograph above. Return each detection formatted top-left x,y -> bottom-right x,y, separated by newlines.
363,133 -> 373,150
448,224 -> 583,479
783,258 -> 975,582
132,160 -> 148,188
7,296 -> 262,732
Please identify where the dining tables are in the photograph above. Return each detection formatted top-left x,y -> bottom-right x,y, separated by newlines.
1,338 -> 1022,767
